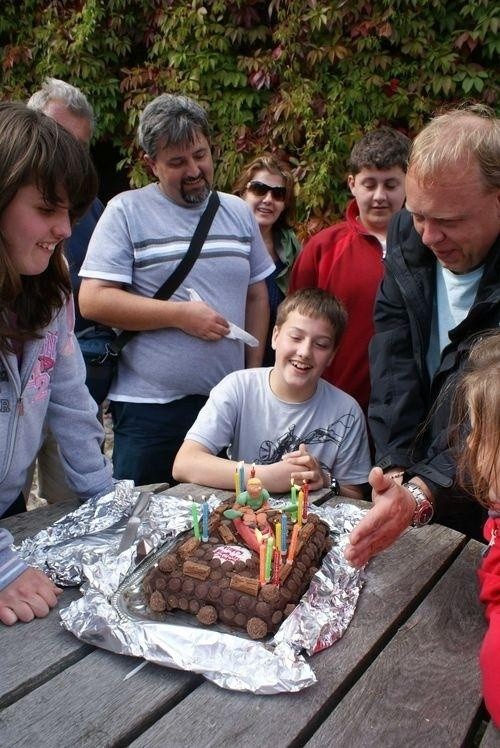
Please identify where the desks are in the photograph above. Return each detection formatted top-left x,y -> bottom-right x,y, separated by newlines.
2,479 -> 499,746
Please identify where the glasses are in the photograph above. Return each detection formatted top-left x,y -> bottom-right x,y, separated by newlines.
246,179 -> 287,203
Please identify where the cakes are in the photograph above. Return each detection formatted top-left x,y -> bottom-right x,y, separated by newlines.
142,479 -> 333,639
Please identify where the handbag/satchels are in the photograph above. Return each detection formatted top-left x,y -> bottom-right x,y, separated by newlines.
77,327 -> 120,405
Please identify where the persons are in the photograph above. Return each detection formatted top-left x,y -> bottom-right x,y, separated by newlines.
344,103 -> 499,569
289,124 -> 410,416
15,76 -> 116,507
455,331 -> 500,732
173,287 -> 372,499
1,107 -> 116,626
79,93 -> 276,487
229,153 -> 302,368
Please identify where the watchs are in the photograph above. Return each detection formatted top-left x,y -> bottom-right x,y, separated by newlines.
404,482 -> 434,529
328,476 -> 341,497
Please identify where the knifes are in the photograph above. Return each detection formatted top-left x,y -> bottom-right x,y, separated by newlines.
111,491 -> 152,558
